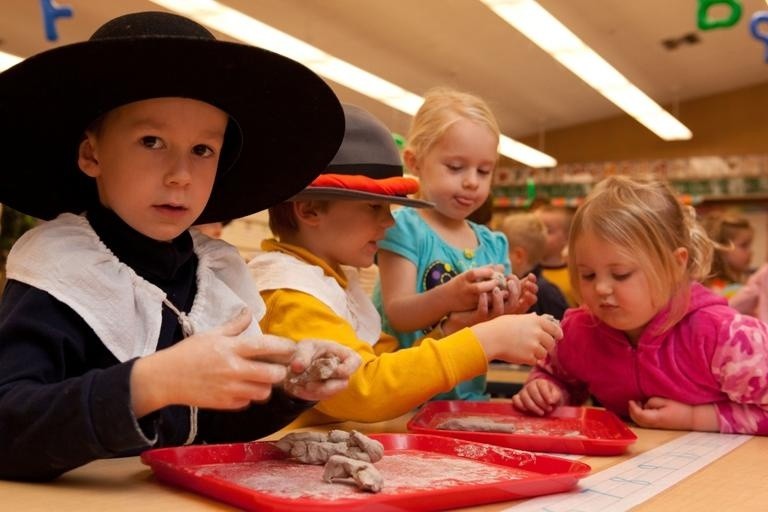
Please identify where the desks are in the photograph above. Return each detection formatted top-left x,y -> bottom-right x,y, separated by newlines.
0,409 -> 768,508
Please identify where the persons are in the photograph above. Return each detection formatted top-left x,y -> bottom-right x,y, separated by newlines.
1,10 -> 365,487
194,220 -> 229,240
369,82 -> 539,400
510,170 -> 768,434
246,102 -> 564,440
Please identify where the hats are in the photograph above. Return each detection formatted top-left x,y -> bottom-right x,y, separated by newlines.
291,102 -> 435,209
1,11 -> 346,227
